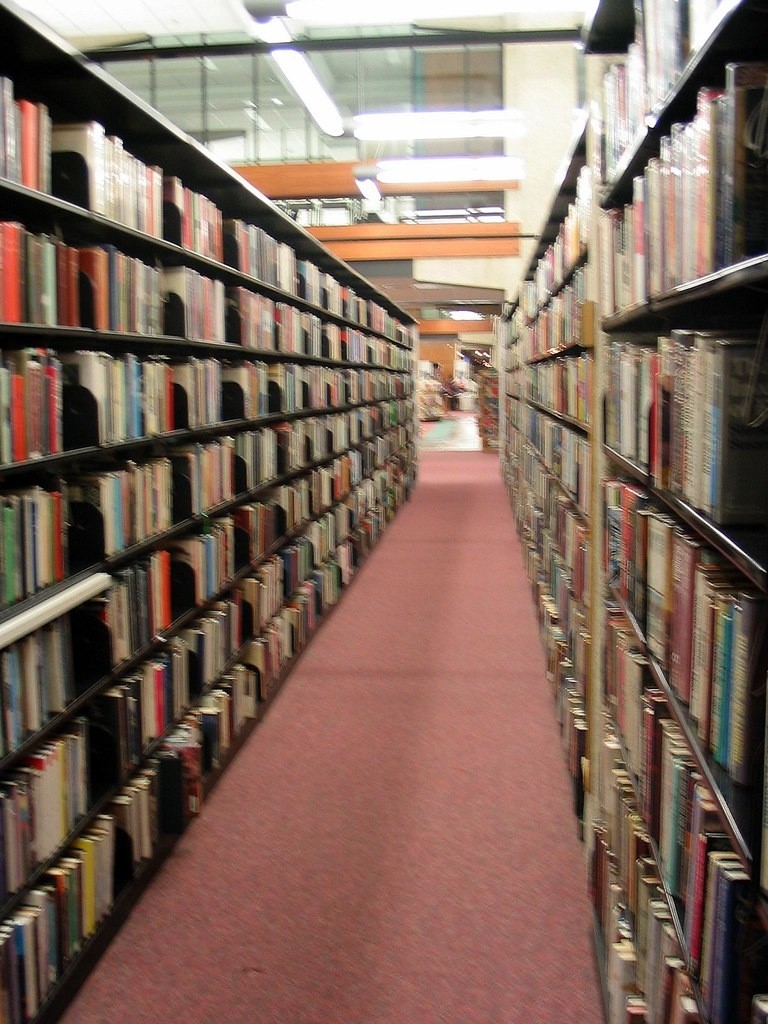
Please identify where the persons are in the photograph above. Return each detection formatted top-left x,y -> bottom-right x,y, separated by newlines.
426,362 -> 466,411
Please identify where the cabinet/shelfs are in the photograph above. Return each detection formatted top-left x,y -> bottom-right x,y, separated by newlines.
497,0 -> 768,1024
0,0 -> 420,1024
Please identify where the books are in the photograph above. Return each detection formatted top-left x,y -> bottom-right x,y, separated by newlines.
468,5 -> 761,1020
4,80 -> 415,1021
